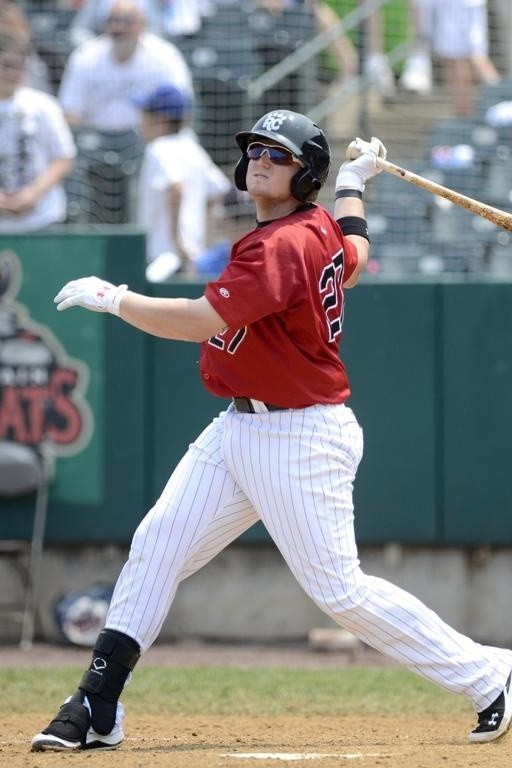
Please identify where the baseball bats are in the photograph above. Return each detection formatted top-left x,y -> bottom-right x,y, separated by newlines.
347,140 -> 512,230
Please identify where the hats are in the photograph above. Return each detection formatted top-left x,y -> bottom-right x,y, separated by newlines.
130,83 -> 188,117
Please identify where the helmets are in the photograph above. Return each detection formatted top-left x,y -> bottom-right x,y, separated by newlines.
234,110 -> 330,201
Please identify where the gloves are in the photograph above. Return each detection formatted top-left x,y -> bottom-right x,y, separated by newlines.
53,276 -> 128,317
335,138 -> 387,193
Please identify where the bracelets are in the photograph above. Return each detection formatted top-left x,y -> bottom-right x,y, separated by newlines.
332,188 -> 363,200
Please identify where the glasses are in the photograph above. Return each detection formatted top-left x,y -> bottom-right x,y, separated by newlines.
246,141 -> 305,169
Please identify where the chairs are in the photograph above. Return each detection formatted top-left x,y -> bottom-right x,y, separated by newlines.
0,426 -> 54,649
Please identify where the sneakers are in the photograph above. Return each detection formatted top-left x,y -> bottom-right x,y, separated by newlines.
469,673 -> 511,742
31,695 -> 124,752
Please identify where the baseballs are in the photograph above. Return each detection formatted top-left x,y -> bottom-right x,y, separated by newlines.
145,252 -> 181,284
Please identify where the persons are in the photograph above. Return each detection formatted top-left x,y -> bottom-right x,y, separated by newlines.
0,2 -> 512,236
54,108 -> 510,754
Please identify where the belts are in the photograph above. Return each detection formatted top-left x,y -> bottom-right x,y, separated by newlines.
234,397 -> 286,413
